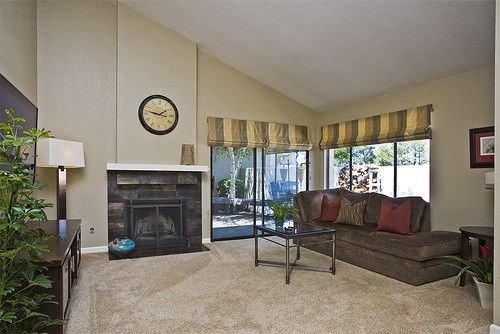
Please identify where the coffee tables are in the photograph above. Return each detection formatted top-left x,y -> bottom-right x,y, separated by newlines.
255,220 -> 336,285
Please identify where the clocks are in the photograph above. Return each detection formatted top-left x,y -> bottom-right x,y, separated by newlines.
138,95 -> 178,135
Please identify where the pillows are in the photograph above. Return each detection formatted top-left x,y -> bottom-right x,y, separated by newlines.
376,199 -> 411,235
319,194 -> 366,226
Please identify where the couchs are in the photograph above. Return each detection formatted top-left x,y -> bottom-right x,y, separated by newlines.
292,187 -> 472,287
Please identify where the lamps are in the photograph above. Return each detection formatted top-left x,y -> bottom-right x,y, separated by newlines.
36,138 -> 85,220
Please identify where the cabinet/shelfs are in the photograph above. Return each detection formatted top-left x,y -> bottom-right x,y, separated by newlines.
2,219 -> 81,334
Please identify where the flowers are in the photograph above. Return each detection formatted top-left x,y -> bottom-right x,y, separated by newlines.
433,244 -> 493,286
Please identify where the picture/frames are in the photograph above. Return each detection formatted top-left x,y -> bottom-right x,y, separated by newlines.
469,126 -> 494,168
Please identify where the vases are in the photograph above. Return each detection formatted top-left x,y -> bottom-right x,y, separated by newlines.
472,276 -> 493,309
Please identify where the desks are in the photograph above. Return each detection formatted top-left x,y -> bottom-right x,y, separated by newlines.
459,226 -> 494,287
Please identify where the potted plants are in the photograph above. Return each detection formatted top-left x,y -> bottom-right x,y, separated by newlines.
267,199 -> 300,226
